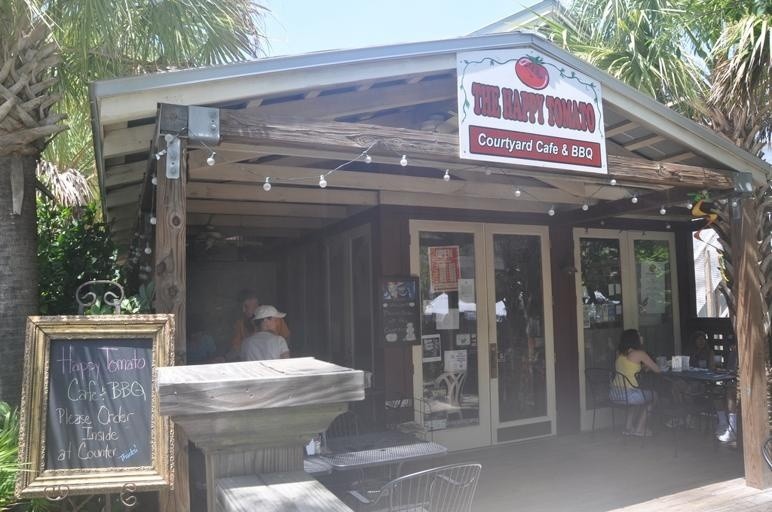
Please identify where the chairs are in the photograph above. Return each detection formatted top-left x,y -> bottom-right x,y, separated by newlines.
583,347 -> 772,473
315,370 -> 484,512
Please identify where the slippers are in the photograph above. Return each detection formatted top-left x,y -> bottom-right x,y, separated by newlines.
622,427 -> 653,437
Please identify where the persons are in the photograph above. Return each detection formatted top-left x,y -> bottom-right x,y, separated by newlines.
663,329 -> 717,430
239,304 -> 291,361
607,328 -> 661,437
718,385 -> 738,441
225,289 -> 290,363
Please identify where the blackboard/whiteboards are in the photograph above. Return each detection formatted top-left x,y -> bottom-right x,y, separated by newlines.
378,275 -> 420,347
13,313 -> 177,501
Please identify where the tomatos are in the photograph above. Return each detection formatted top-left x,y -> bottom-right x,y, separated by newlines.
516,58 -> 549,90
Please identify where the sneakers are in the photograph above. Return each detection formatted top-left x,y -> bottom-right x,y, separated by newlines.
718,431 -> 737,442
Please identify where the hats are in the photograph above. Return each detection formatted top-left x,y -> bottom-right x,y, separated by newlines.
251,305 -> 287,321
